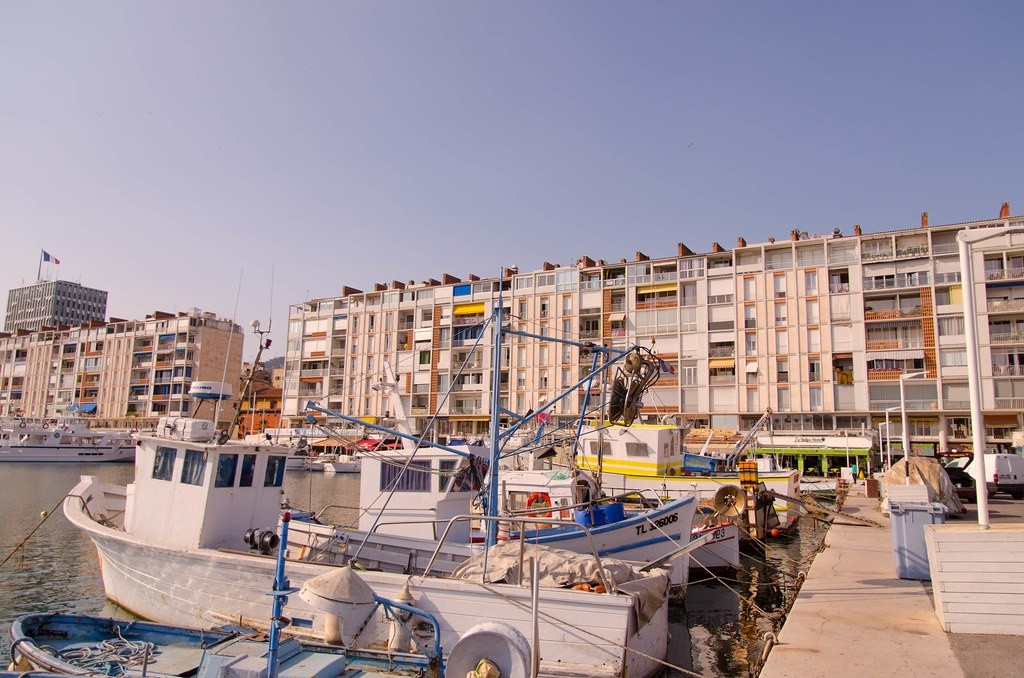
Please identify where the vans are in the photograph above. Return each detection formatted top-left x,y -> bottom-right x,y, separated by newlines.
944,453 -> 1024,500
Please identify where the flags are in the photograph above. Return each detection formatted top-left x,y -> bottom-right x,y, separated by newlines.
42,250 -> 60,265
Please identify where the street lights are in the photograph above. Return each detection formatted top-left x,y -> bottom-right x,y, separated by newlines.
878,421 -> 892,473
886,406 -> 902,469
956,226 -> 1024,530
899,371 -> 929,485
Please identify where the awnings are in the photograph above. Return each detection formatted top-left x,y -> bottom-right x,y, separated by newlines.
69,402 -> 97,412
291,436 -> 381,450
709,359 -> 736,369
608,313 -> 626,322
454,303 -> 484,315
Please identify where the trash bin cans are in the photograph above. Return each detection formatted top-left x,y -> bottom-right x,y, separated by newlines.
889,501 -> 949,581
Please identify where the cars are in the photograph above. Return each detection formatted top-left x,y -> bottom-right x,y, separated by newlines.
934,451 -> 976,504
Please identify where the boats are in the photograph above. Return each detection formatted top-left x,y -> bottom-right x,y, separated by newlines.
62,267 -> 670,678
8,512 -> 448,678
285,436 -> 362,473
280,361 -> 700,606
498,419 -> 801,537
469,469 -> 741,567
0,416 -> 136,462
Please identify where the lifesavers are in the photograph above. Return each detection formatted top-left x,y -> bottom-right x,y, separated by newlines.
526,493 -> 551,521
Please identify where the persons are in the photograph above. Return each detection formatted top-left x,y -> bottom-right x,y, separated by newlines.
851,462 -> 857,484
